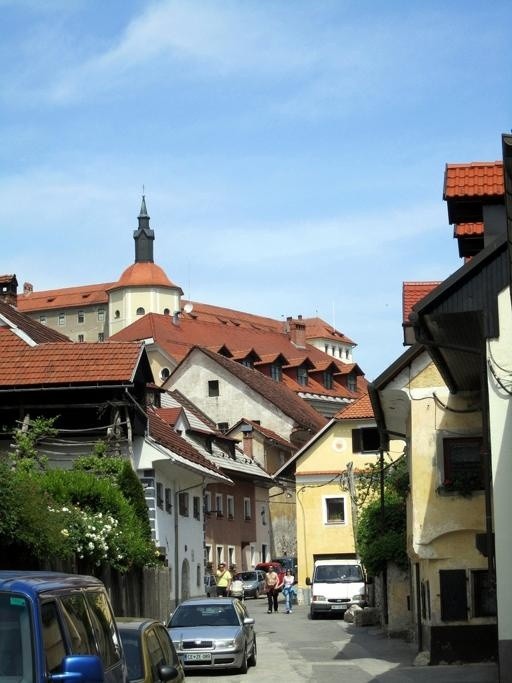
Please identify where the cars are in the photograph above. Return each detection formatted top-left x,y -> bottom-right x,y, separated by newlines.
163,595 -> 258,675
113,614 -> 186,682
229,557 -> 297,598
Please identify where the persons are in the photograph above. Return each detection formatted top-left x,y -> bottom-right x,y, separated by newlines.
225,574 -> 246,602
263,565 -> 281,613
276,568 -> 295,614
216,563 -> 232,597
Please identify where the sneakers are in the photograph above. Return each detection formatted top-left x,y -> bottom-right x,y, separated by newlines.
275,611 -> 278,613
267,610 -> 272,613
291,608 -> 295,612
287,610 -> 289,614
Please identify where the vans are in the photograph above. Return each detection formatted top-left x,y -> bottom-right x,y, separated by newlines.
1,566 -> 130,682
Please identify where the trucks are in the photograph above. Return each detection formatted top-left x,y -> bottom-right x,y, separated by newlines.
304,558 -> 369,620
